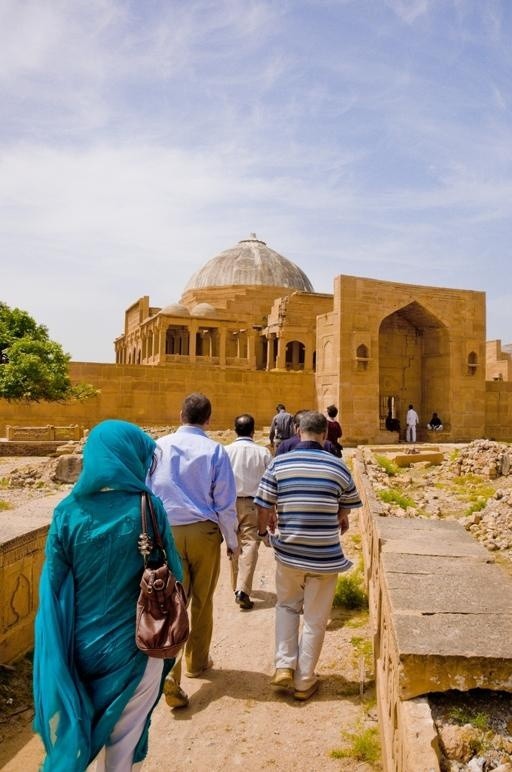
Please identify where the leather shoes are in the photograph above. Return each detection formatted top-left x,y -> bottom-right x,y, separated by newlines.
235,591 -> 254,610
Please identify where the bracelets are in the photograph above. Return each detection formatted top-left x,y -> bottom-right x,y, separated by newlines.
258,530 -> 268,537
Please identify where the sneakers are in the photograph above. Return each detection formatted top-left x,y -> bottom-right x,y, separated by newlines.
270,669 -> 294,693
184,657 -> 213,678
293,681 -> 319,699
162,677 -> 188,709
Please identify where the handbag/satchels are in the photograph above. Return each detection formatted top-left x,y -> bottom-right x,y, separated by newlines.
134,562 -> 190,659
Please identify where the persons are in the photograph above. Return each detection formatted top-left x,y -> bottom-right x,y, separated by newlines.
385,411 -> 400,433
143,390 -> 240,709
218,413 -> 274,609
29,417 -> 187,772
404,404 -> 419,443
268,403 -> 295,457
426,412 -> 444,431
275,409 -> 338,457
252,412 -> 363,702
324,404 -> 343,448
